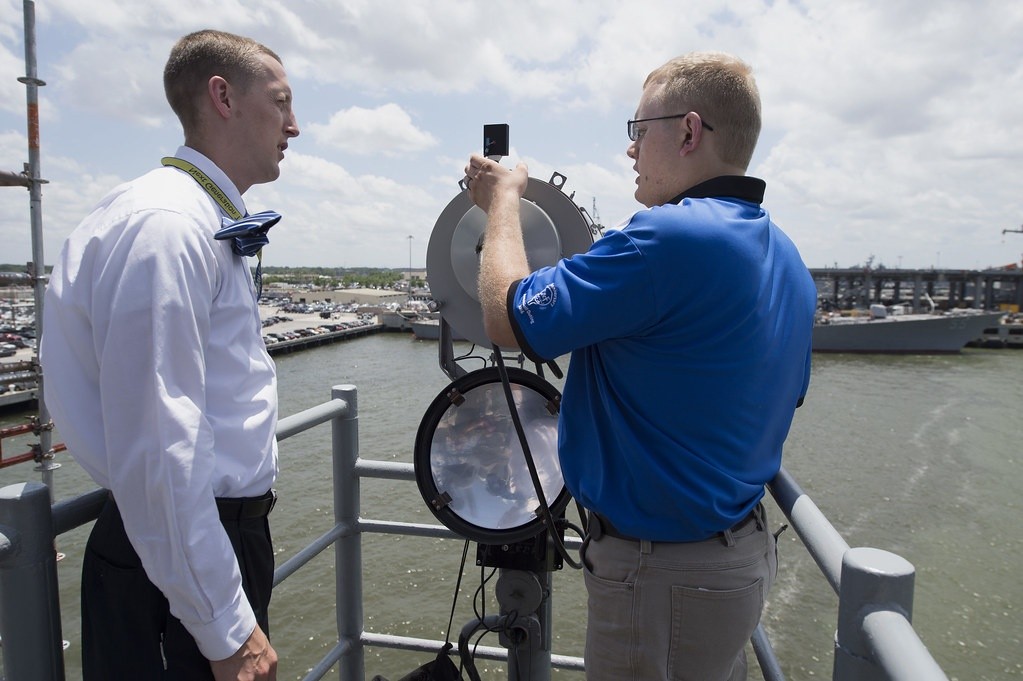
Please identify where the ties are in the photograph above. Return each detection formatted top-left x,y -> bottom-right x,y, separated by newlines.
213,210 -> 282,257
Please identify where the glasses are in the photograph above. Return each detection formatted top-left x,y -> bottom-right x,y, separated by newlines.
627,114 -> 715,142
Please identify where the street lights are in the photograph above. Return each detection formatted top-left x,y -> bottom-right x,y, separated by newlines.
406,235 -> 414,308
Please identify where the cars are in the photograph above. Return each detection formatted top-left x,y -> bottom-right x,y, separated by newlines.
261,296 -> 401,344
0,300 -> 36,355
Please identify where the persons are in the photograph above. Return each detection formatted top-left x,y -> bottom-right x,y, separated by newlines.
463,49 -> 818,681
38,29 -> 300,681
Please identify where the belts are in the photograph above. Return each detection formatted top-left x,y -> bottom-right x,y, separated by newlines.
215,487 -> 278,521
585,506 -> 760,545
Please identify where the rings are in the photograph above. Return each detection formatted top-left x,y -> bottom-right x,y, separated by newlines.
475,171 -> 484,179
467,179 -> 471,190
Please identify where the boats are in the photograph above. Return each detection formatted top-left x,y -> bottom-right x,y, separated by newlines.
411,307 -> 1012,352
